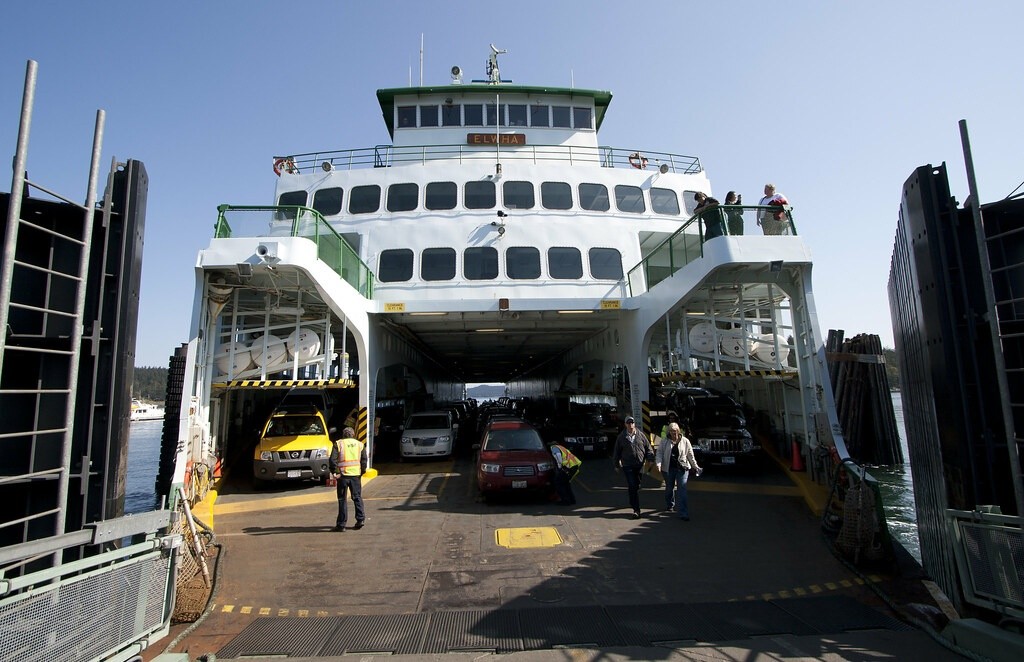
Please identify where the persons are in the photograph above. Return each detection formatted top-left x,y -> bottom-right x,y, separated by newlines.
694,192 -> 723,241
551,445 -> 581,484
329,427 -> 367,532
614,416 -> 655,518
723,191 -> 744,235
757,184 -> 789,235
660,413 -> 685,439
655,423 -> 702,521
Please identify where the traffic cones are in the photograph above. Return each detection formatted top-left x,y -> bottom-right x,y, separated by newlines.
790,433 -> 806,473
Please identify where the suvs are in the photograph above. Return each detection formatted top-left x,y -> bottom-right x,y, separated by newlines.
681,393 -> 753,475
251,401 -> 333,489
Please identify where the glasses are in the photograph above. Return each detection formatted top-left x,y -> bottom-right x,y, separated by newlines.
626,421 -> 635,424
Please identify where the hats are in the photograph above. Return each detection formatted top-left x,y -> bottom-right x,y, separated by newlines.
625,415 -> 635,423
667,423 -> 680,431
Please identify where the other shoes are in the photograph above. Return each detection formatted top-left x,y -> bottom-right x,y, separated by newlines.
352,519 -> 365,530
679,516 -> 690,522
329,525 -> 346,532
666,506 -> 674,512
632,511 -> 640,519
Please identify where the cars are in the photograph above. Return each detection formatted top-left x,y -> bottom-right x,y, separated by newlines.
472,421 -> 555,492
545,413 -> 611,459
439,394 -> 617,442
276,388 -> 335,425
396,411 -> 460,460
614,365 -> 749,437
